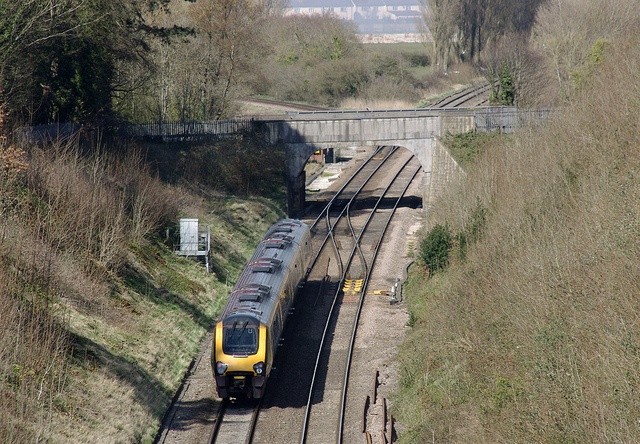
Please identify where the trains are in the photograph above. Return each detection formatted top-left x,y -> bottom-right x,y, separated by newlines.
210,219 -> 312,404
